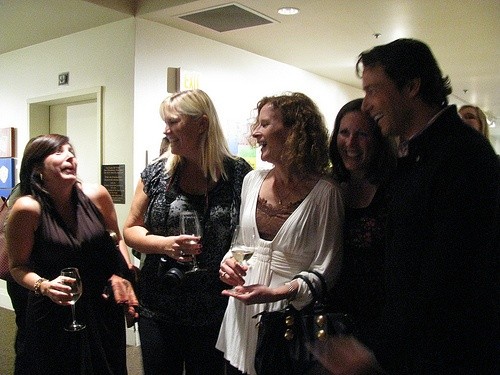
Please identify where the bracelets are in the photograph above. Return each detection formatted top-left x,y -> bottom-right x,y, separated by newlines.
285,282 -> 295,301
33,278 -> 48,297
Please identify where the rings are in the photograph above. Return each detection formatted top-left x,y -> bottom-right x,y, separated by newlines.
221,272 -> 227,278
181,251 -> 186,257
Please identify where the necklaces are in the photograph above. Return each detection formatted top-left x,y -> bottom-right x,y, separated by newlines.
273,173 -> 306,206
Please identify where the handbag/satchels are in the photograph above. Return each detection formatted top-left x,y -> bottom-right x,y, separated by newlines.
251,270 -> 355,375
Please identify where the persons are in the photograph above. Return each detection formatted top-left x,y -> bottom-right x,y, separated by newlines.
330,98 -> 409,321
306,39 -> 500,375
2,177 -> 125,355
202,92 -> 341,375
123,89 -> 253,375
456,103 -> 490,142
8,133 -> 140,375
